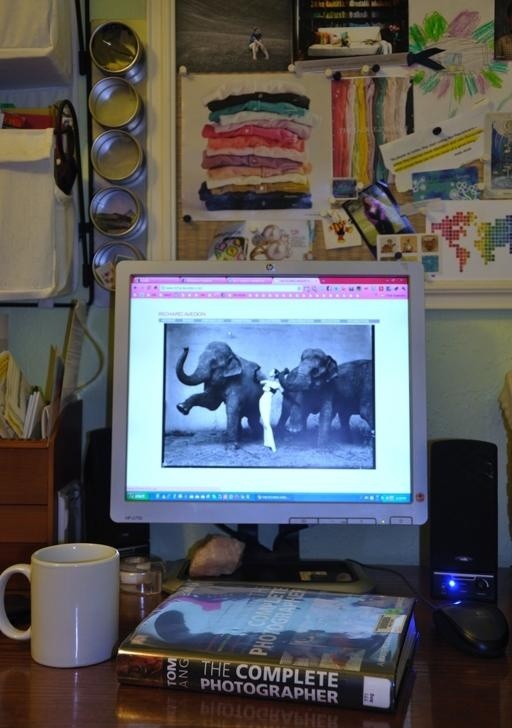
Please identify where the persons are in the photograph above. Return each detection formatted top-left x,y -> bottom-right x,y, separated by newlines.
250,29 -> 268,61
257,368 -> 284,451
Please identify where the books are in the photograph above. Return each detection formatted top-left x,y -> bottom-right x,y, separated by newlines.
114,578 -> 422,718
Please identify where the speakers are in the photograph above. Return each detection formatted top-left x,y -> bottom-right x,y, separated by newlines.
429,439 -> 498,603
84,428 -> 150,559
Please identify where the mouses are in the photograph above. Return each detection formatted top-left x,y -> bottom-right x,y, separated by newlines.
433,599 -> 509,656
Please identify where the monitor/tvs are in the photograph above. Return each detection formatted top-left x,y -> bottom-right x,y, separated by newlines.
109,260 -> 428,595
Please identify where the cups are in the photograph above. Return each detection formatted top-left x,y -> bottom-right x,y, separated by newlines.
0,541 -> 119,670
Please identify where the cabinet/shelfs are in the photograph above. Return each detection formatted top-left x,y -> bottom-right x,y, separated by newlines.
0,397 -> 85,595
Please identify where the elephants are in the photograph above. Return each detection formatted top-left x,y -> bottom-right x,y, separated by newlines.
175,339 -> 266,451
270,346 -> 376,448
